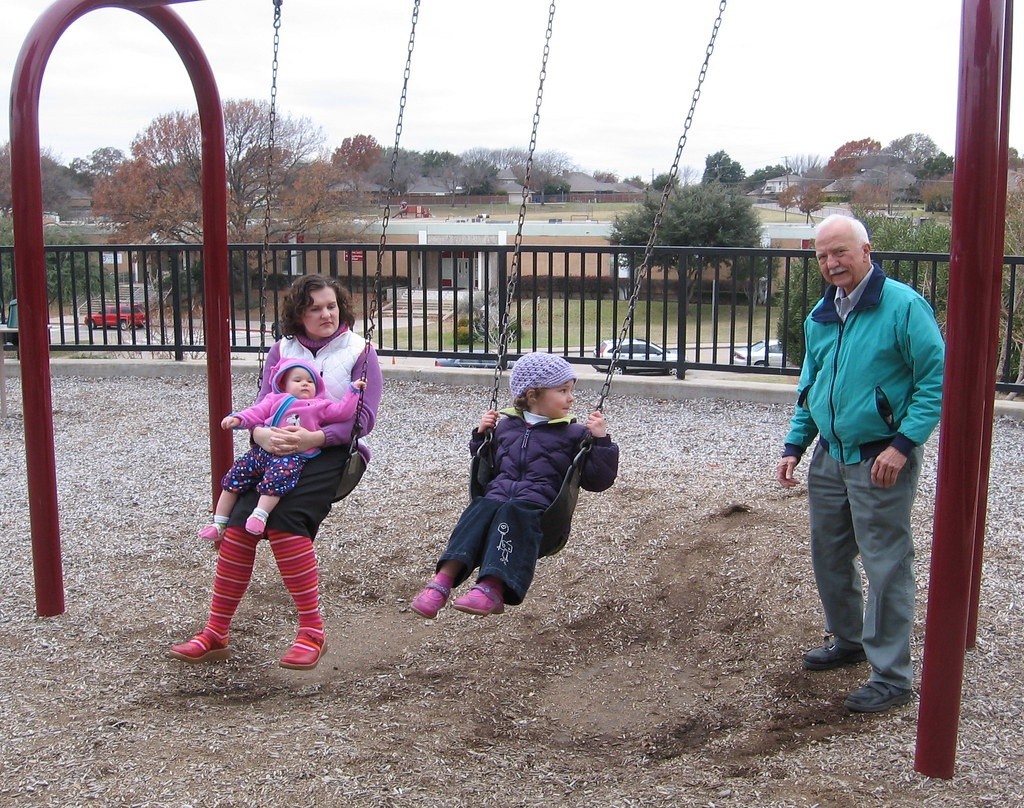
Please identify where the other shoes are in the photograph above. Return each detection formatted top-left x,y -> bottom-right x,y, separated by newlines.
246,508 -> 269,535
411,582 -> 450,619
454,582 -> 505,615
279,630 -> 328,671
169,629 -> 230,664
199,515 -> 229,541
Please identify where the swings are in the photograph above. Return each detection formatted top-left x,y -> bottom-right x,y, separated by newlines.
249,0 -> 423,497
470,0 -> 729,557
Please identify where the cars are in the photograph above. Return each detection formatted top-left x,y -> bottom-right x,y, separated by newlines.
732,338 -> 796,366
434,350 -> 516,369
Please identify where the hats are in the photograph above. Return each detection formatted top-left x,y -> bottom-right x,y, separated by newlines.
509,352 -> 579,402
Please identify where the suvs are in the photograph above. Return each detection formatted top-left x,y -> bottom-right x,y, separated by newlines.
84,303 -> 146,331
591,339 -> 689,377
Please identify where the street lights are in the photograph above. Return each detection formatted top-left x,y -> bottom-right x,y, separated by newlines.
764,170 -> 789,189
860,169 -> 893,216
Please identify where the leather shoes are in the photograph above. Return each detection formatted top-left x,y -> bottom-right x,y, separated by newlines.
803,642 -> 867,670
844,680 -> 912,712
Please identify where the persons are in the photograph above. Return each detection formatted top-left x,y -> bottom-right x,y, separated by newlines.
168,273 -> 382,671
411,352 -> 619,619
198,358 -> 368,541
778,214 -> 945,712
